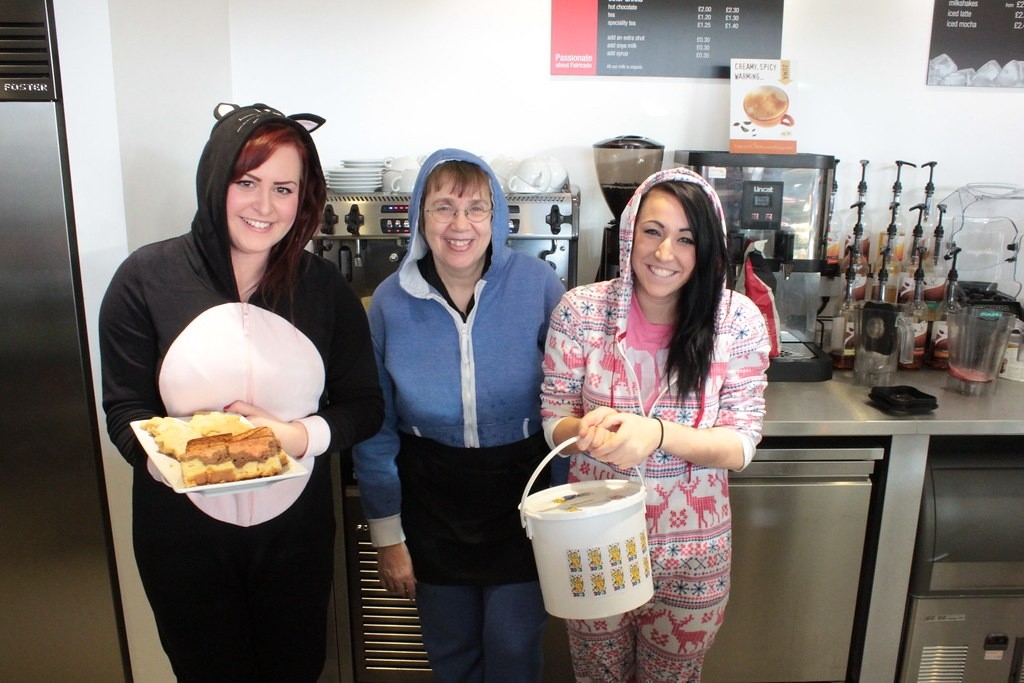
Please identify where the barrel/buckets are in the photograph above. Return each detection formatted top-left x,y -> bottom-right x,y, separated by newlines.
518,435 -> 654,619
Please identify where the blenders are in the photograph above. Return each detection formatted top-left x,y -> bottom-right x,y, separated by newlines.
593,135 -> 665,282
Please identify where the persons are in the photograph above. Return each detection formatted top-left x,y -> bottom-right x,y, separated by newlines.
351,148 -> 570,683
540,168 -> 771,683
98,104 -> 388,683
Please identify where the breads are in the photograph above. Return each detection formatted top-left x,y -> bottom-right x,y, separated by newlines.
140,410 -> 289,488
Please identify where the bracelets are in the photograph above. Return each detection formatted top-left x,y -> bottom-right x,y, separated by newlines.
656,418 -> 663,449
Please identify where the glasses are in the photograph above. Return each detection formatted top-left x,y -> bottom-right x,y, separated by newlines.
421,202 -> 494,223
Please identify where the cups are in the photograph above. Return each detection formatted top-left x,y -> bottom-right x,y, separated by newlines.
943,309 -> 1016,396
480,154 -> 567,193
381,153 -> 429,193
852,301 -> 914,387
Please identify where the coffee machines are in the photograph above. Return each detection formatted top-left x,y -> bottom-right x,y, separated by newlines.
672,150 -> 839,383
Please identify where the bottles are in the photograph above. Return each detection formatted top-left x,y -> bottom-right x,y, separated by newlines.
827,161 -> 963,370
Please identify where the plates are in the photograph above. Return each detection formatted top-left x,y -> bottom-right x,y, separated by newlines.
324,159 -> 385,194
130,412 -> 307,497
